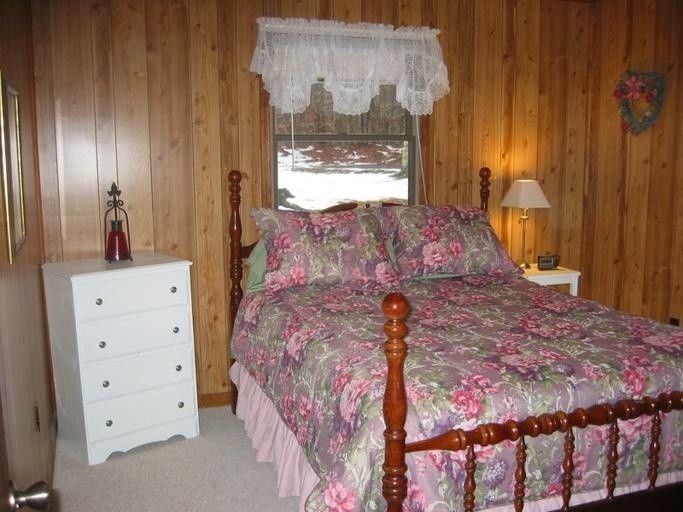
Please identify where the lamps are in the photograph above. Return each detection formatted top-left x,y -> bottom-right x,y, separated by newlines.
500,179 -> 551,268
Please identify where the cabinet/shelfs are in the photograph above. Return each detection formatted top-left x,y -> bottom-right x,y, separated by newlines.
41,251 -> 201,466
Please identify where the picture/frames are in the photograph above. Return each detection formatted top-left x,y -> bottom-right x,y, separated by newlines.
1,73 -> 27,264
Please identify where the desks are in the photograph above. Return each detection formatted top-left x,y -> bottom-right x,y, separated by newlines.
521,263 -> 582,297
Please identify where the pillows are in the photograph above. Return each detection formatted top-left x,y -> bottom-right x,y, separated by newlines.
242,206 -> 526,296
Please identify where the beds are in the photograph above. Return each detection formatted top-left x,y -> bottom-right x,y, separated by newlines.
229,168 -> 682,511
